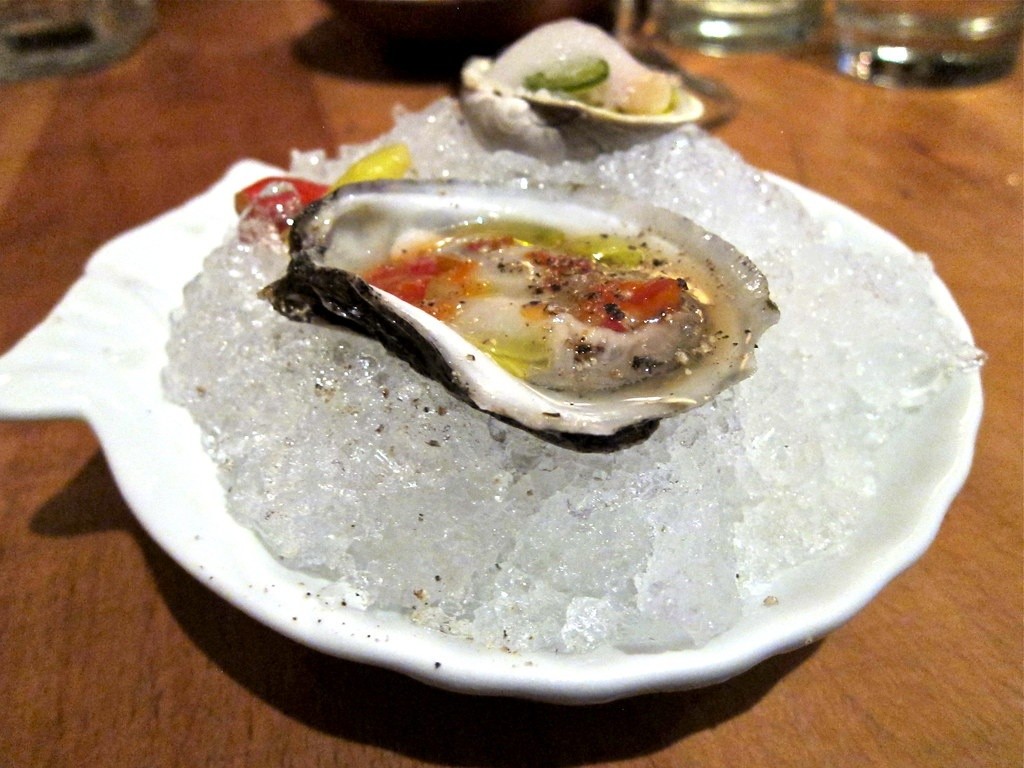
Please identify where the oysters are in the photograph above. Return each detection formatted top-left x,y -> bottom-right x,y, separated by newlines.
255,178 -> 781,455
457,18 -> 707,165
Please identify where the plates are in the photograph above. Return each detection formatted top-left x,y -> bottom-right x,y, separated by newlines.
0,157 -> 986,708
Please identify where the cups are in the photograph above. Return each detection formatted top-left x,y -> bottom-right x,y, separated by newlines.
648,2 -> 804,56
832,1 -> 1023,89
